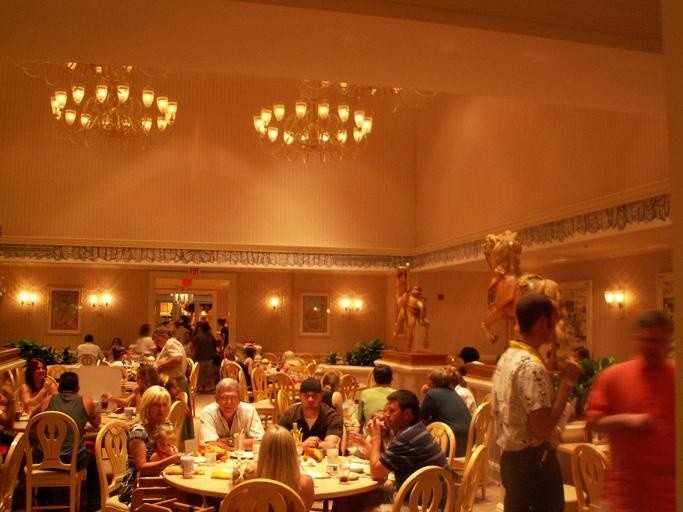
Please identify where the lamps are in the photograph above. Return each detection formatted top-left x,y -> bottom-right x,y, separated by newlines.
16,56 -> 180,138
252,78 -> 437,166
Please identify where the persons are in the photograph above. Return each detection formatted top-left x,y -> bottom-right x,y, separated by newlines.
9,314 -> 476,512
490,292 -> 582,512
584,310 -> 677,512
575,346 -> 589,362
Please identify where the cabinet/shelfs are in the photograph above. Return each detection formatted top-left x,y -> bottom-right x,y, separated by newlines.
234,344 -> 263,391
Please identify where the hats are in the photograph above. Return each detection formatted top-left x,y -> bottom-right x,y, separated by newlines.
300,378 -> 322,394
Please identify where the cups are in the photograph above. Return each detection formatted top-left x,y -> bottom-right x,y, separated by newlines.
180,423 -> 369,484
15,401 -> 135,423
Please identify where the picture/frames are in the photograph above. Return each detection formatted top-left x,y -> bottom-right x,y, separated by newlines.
654,272 -> 678,367
45,283 -> 83,337
299,292 -> 331,339
550,279 -> 595,363
